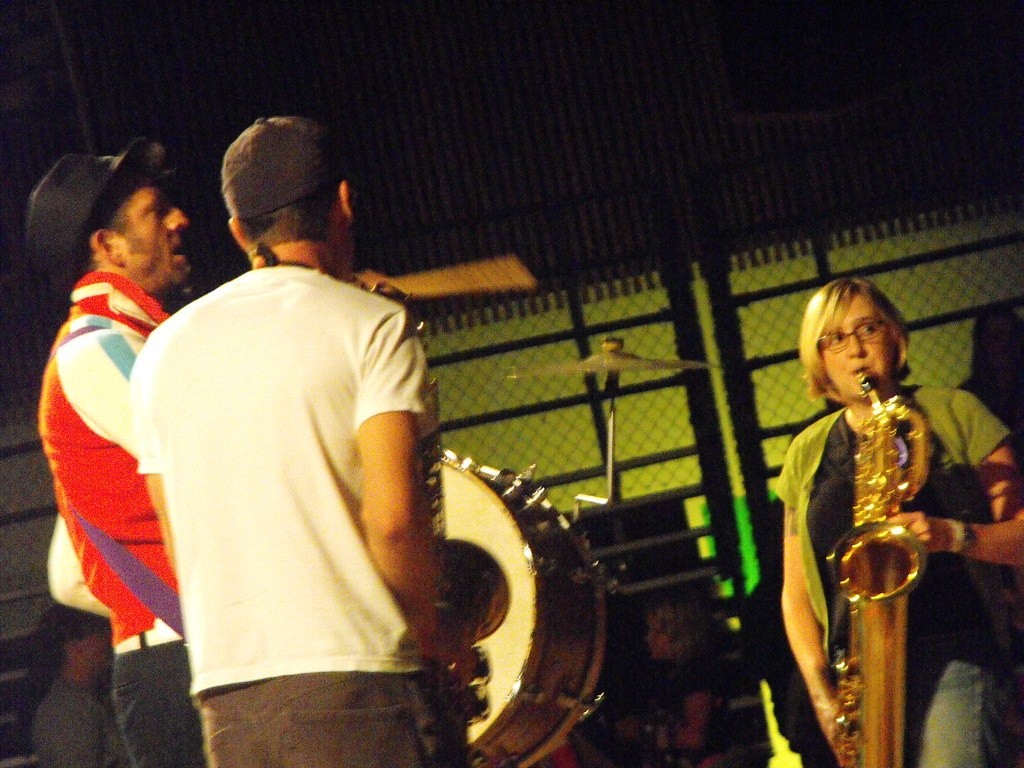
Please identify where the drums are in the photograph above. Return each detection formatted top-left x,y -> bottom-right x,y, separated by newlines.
439,445 -> 612,768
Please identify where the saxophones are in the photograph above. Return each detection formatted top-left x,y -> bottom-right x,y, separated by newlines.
829,368 -> 930,768
353,276 -> 511,768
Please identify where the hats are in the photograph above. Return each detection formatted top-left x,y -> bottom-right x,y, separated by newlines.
23,134 -> 151,326
221,116 -> 338,219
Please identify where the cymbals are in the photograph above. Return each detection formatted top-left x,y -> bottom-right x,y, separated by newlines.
506,352 -> 728,380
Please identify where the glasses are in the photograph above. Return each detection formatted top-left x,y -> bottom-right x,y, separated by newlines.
818,318 -> 889,350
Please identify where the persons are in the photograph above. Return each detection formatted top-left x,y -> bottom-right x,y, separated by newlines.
766,275 -> 1023,768
20,139 -> 194,768
612,583 -> 730,768
126,114 -> 484,768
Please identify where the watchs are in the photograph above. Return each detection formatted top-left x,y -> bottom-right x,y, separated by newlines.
956,519 -> 977,559
